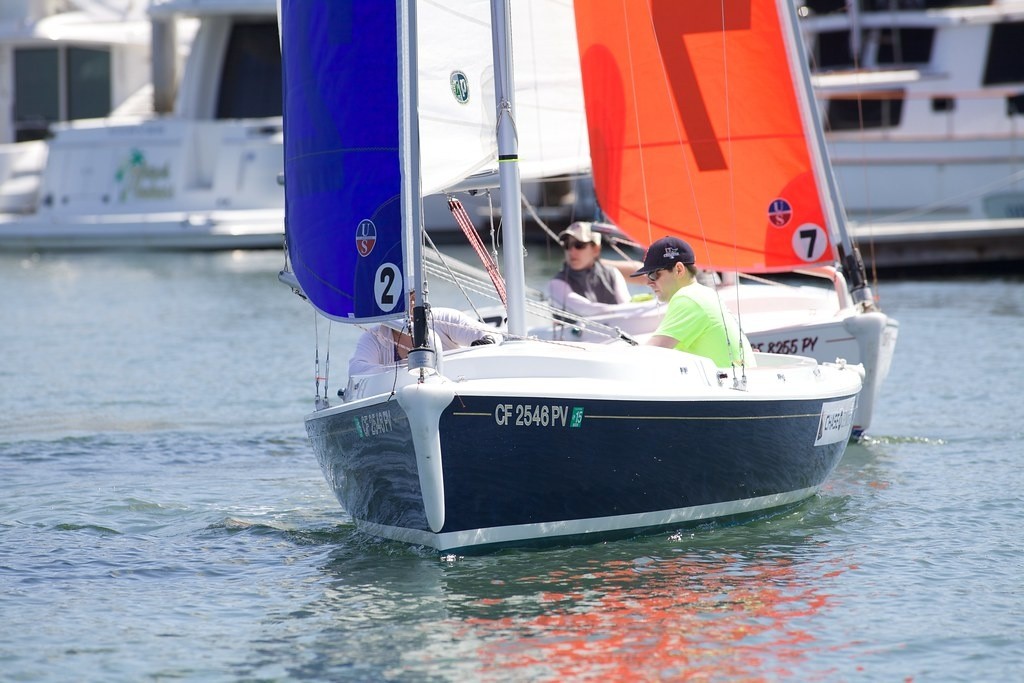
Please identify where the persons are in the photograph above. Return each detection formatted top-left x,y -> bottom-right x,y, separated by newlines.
629,235 -> 756,368
348,292 -> 502,376
548,223 -> 630,326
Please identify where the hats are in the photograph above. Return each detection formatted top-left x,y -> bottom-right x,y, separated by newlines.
630,236 -> 695,277
409,276 -> 429,293
559,222 -> 602,245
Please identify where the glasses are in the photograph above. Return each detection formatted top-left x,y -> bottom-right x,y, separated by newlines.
647,268 -> 673,281
563,240 -> 592,250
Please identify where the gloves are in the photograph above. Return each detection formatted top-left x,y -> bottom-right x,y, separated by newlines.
471,335 -> 495,346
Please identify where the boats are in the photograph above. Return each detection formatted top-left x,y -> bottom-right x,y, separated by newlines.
1,1 -> 1024,264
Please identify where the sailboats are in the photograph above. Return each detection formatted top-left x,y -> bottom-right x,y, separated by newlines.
276,1 -> 899,560
